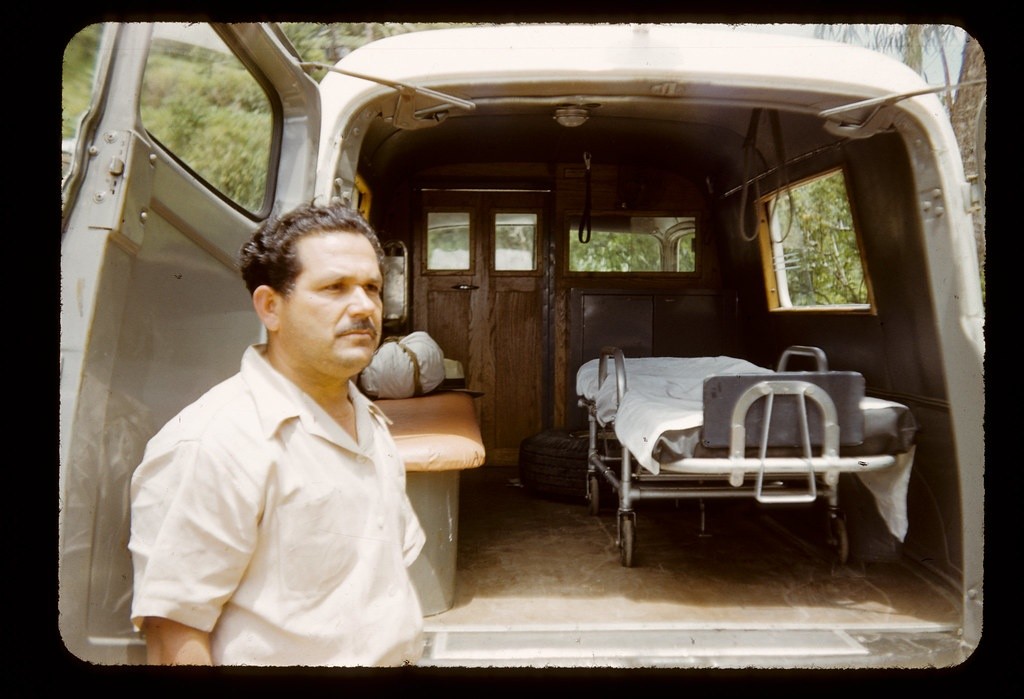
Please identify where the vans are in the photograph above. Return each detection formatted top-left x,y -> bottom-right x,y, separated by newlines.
54,21 -> 985,667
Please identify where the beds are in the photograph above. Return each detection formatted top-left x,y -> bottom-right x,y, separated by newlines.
576,346 -> 922,567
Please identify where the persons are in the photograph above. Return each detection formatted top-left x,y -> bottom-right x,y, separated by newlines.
129,205 -> 425,667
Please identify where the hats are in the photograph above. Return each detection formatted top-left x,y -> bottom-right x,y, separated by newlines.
434,358 -> 486,397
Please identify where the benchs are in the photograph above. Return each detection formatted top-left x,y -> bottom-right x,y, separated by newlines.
371,390 -> 486,616
